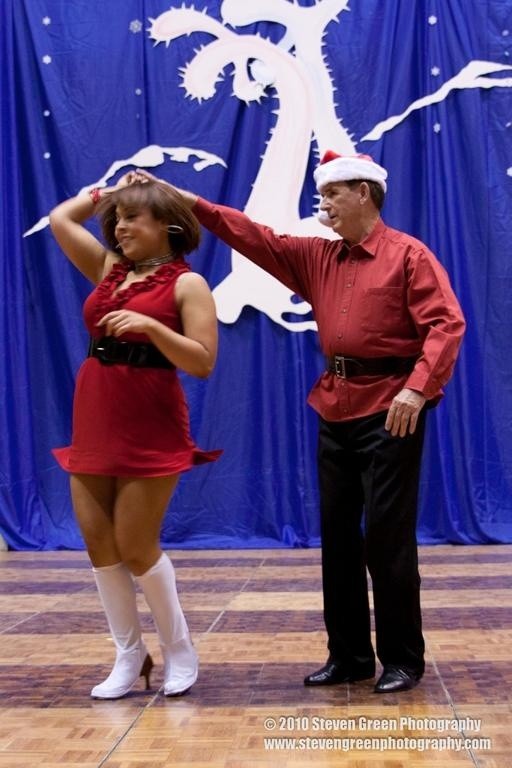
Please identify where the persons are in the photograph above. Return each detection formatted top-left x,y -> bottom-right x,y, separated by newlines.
49,169 -> 220,700
133,151 -> 467,687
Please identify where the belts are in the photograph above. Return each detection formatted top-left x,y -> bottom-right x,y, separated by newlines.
86,335 -> 177,373
325,356 -> 414,378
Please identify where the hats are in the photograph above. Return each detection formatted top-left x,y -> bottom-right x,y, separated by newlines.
312,149 -> 390,193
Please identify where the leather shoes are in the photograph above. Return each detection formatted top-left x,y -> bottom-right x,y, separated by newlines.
303,656 -> 376,687
374,664 -> 425,694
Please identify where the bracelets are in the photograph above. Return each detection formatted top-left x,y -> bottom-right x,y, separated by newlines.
88,185 -> 101,205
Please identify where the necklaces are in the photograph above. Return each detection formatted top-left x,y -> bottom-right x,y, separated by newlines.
132,247 -> 178,268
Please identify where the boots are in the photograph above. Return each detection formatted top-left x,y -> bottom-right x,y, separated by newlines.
89,562 -> 155,702
129,548 -> 201,698
